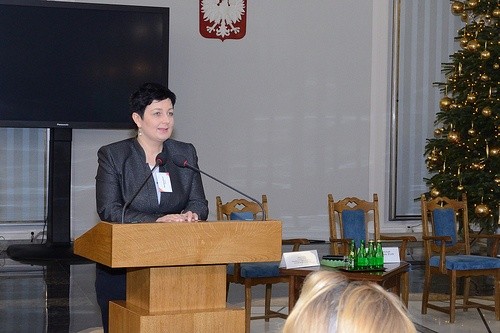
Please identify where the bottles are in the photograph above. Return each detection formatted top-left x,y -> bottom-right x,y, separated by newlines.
375,239 -> 383,269
366,239 -> 376,269
356,239 -> 368,270
348,239 -> 359,271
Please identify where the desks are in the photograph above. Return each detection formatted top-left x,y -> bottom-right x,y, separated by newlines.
280,263 -> 409,316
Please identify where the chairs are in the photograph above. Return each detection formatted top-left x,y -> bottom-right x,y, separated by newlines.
328,193 -> 417,262
216,195 -> 309,333
421,193 -> 500,323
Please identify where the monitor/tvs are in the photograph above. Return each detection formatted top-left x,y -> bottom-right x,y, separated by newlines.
0,0 -> 170,130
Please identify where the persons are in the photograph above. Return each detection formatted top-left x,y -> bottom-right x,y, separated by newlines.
282,270 -> 417,333
94,82 -> 210,333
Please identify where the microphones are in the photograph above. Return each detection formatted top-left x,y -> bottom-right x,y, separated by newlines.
173,154 -> 266,221
122,153 -> 167,224
